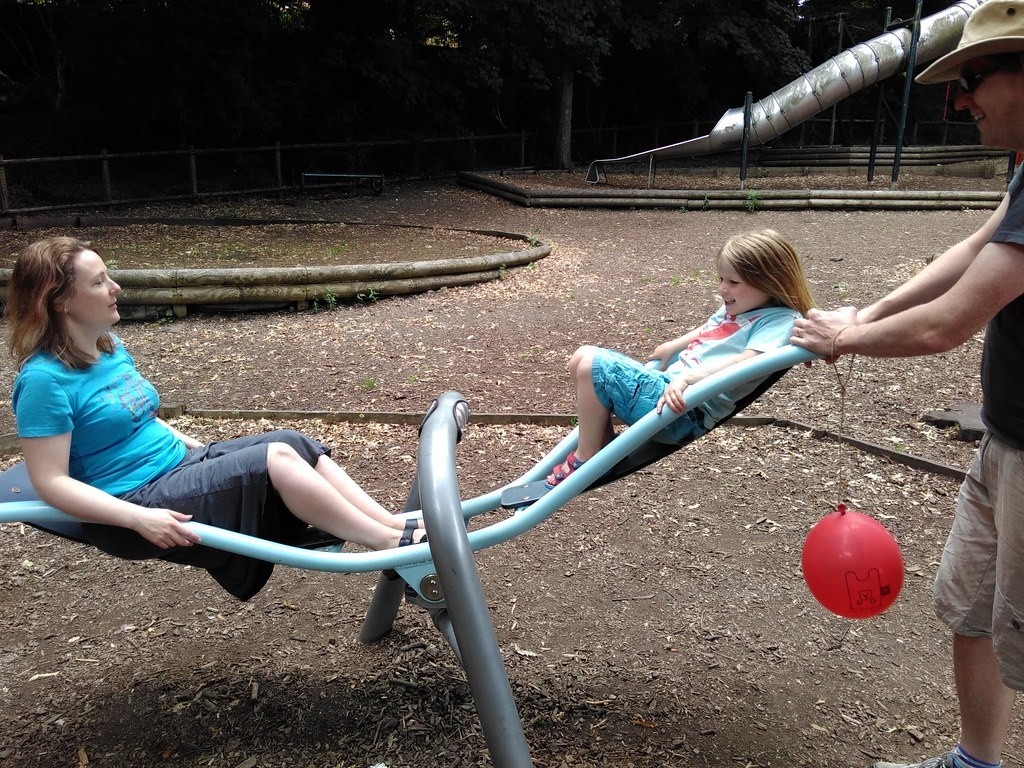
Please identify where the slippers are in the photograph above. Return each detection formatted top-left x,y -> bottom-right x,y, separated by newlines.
382,519 -> 428,581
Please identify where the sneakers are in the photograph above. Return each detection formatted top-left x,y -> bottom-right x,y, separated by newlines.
872,748 -> 961,768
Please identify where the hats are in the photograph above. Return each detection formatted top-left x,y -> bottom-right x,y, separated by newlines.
914,0 -> 1024,84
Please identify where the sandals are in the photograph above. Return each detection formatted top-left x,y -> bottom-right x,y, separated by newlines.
544,451 -> 585,489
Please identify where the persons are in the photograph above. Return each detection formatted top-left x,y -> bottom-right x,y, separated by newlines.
790,0 -> 1023,768
544,227 -> 821,488
5,237 -> 429,601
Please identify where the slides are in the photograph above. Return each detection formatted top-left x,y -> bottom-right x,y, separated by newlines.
584,0 -> 996,186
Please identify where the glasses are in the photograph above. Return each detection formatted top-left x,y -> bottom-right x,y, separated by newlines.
957,64 -> 1004,94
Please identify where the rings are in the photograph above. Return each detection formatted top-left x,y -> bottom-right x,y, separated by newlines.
795,328 -> 802,337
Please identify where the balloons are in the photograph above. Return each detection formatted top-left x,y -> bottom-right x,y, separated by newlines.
801,504 -> 905,619
